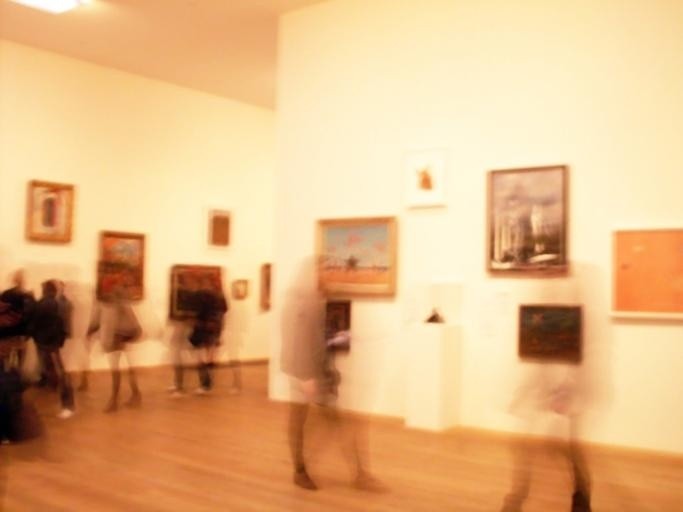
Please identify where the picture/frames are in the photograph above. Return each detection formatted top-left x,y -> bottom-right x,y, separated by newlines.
310,213 -> 401,302
314,296 -> 355,353
230,276 -> 250,302
205,211 -> 235,249
23,180 -> 79,245
163,261 -> 229,321
483,165 -> 573,280
603,226 -> 682,323
512,300 -> 589,367
93,228 -> 150,305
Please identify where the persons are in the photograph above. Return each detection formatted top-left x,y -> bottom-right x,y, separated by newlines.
0,253 -> 619,512
